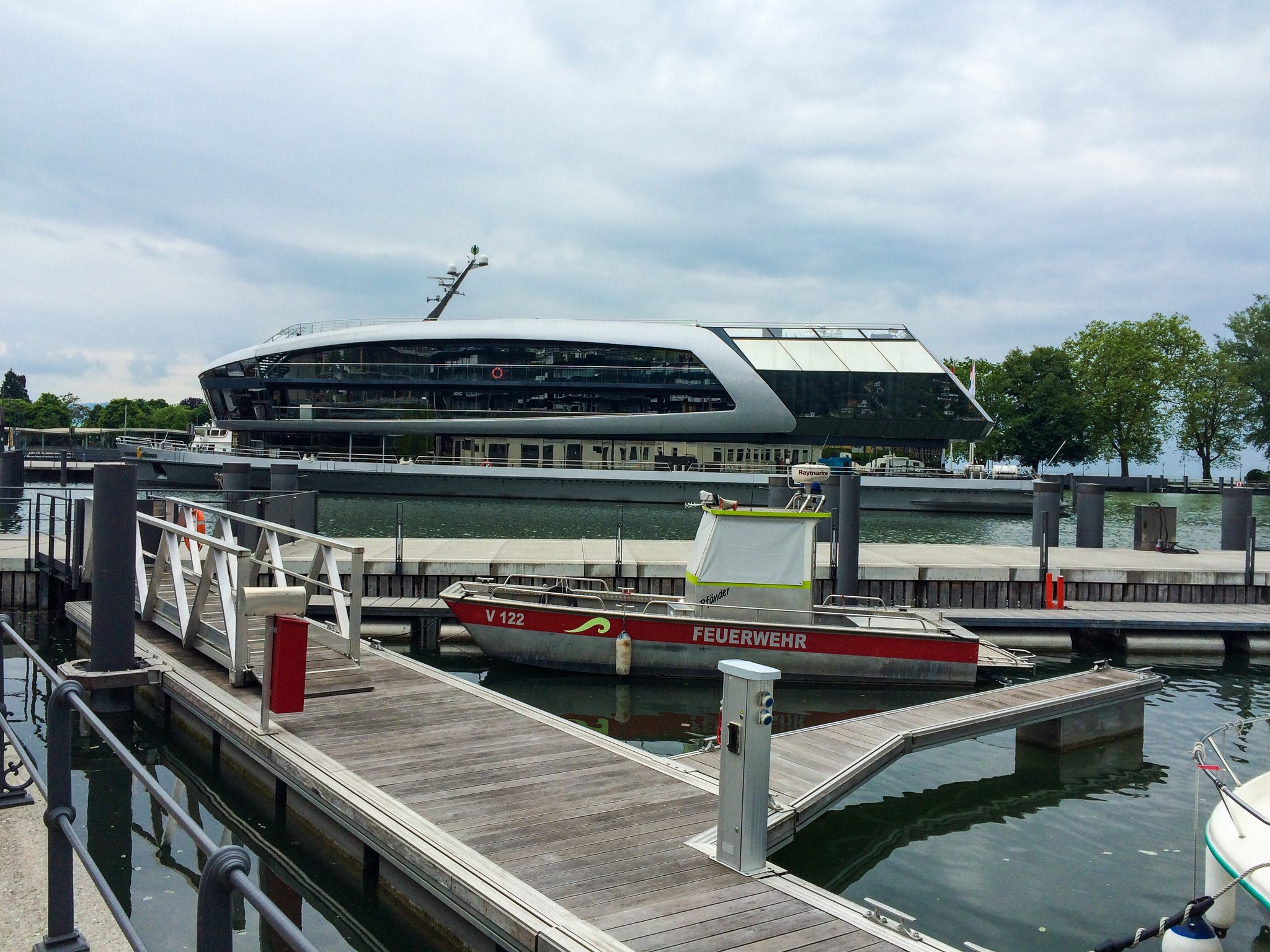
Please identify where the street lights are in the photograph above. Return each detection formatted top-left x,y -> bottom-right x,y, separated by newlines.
1267,465 -> 1270,472
1108,461 -> 1110,477
1162,462 -> 1165,476
1183,463 -> 1186,476
1239,463 -> 1243,482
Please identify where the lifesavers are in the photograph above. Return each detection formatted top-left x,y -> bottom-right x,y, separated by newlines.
480,461 -> 494,467
539,586 -> 578,607
492,368 -> 503,378
184,508 -> 206,552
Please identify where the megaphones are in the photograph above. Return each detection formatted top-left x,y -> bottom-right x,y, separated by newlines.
718,497 -> 737,510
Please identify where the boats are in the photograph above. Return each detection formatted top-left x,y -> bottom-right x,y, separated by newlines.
1194,714 -> 1270,919
115,401 -> 1072,518
438,433 -> 1039,686
